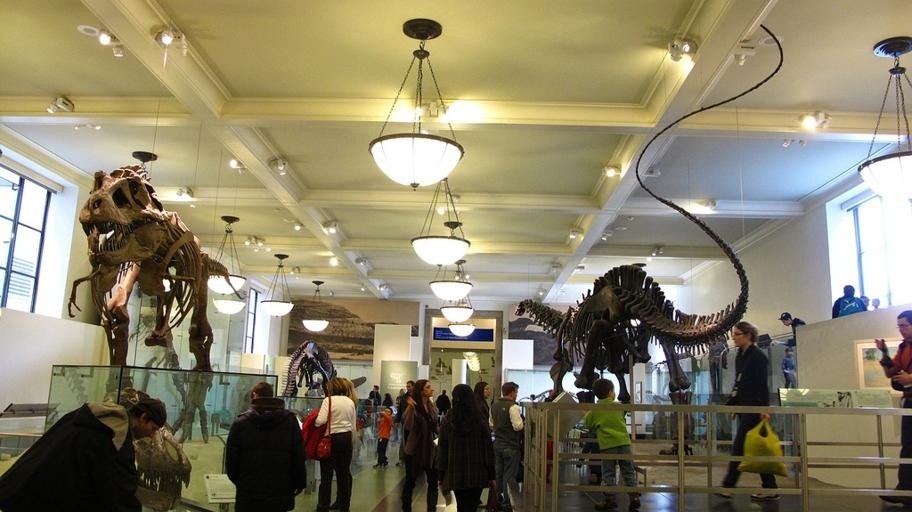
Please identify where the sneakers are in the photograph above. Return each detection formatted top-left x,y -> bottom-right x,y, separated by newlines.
751,493 -> 781,502
879,496 -> 912,505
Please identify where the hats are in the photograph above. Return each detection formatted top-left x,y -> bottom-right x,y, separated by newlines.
127,398 -> 166,426
778,312 -> 791,320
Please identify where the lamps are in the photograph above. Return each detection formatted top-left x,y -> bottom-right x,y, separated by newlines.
144,23 -> 192,69
794,100 -> 839,136
653,32 -> 707,77
44,93 -> 77,117
367,17 -> 469,196
726,42 -> 756,69
410,177 -> 477,272
594,161 -> 628,186
77,23 -> 128,65
857,32 -> 911,205
428,259 -> 477,338
171,144 -> 341,334
674,192 -> 724,217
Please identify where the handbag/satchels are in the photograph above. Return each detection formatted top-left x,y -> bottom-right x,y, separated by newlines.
317,437 -> 332,465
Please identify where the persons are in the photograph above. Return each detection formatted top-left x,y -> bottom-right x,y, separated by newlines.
225,382 -> 307,512
832,286 -> 867,319
314,378 -> 357,512
371,378 -> 524,512
780,313 -> 805,343
716,321 -> 783,501
875,311 -> 912,505
0,387 -> 166,512
782,346 -> 796,389
583,379 -> 640,510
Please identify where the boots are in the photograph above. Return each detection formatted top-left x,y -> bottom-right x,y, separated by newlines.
629,492 -> 641,512
595,495 -> 617,510
